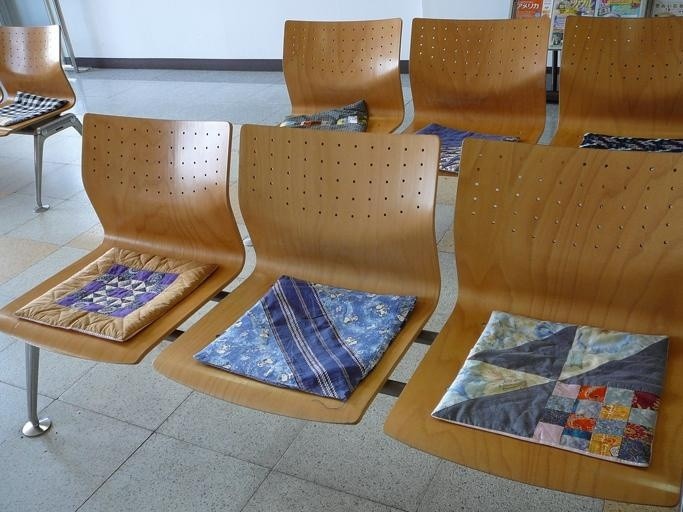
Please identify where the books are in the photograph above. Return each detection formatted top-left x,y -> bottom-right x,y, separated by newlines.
512,0 -> 682,50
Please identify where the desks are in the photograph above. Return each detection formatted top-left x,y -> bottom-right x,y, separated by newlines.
545,44 -> 563,105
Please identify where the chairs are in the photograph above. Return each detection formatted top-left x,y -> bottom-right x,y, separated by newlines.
401,16 -> 551,176
152,123 -> 440,425
547,15 -> 683,148
384,137 -> 683,508
267,18 -> 405,134
0,113 -> 246,439
0,25 -> 85,213
